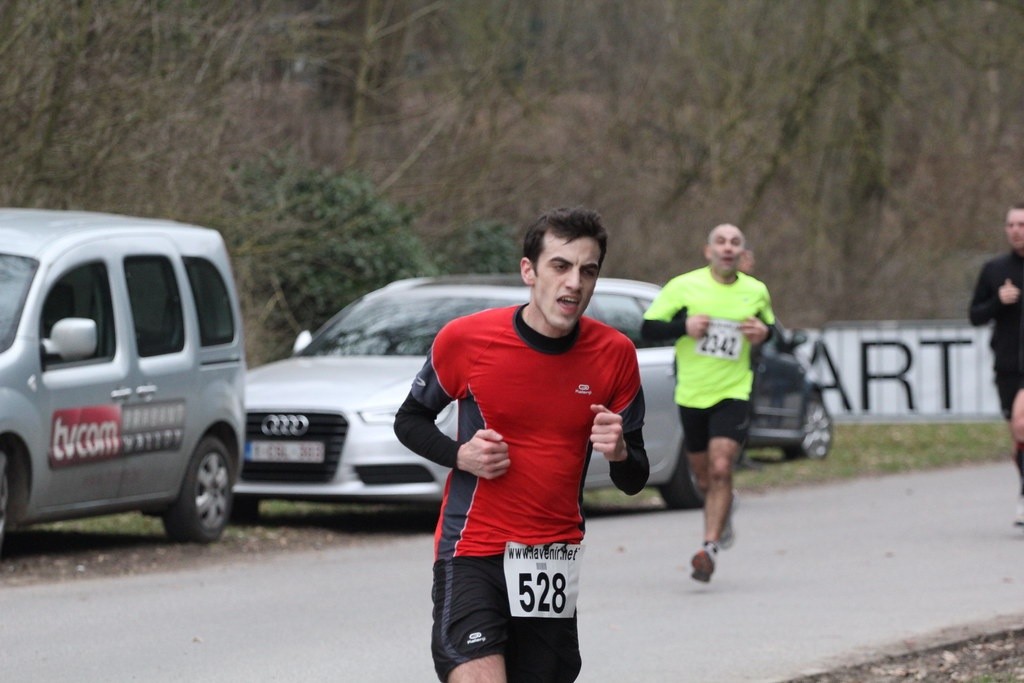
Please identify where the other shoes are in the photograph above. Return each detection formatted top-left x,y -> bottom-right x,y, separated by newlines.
1014,515 -> 1024,526
719,521 -> 735,550
691,542 -> 718,581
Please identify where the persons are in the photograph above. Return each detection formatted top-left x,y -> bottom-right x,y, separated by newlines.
640,224 -> 777,582
968,201 -> 1024,526
393,205 -> 650,683
737,248 -> 758,274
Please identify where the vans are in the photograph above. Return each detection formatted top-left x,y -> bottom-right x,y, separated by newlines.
0,204 -> 247,550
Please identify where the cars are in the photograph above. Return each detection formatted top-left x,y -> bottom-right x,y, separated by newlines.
232,274 -> 707,523
745,318 -> 835,460
